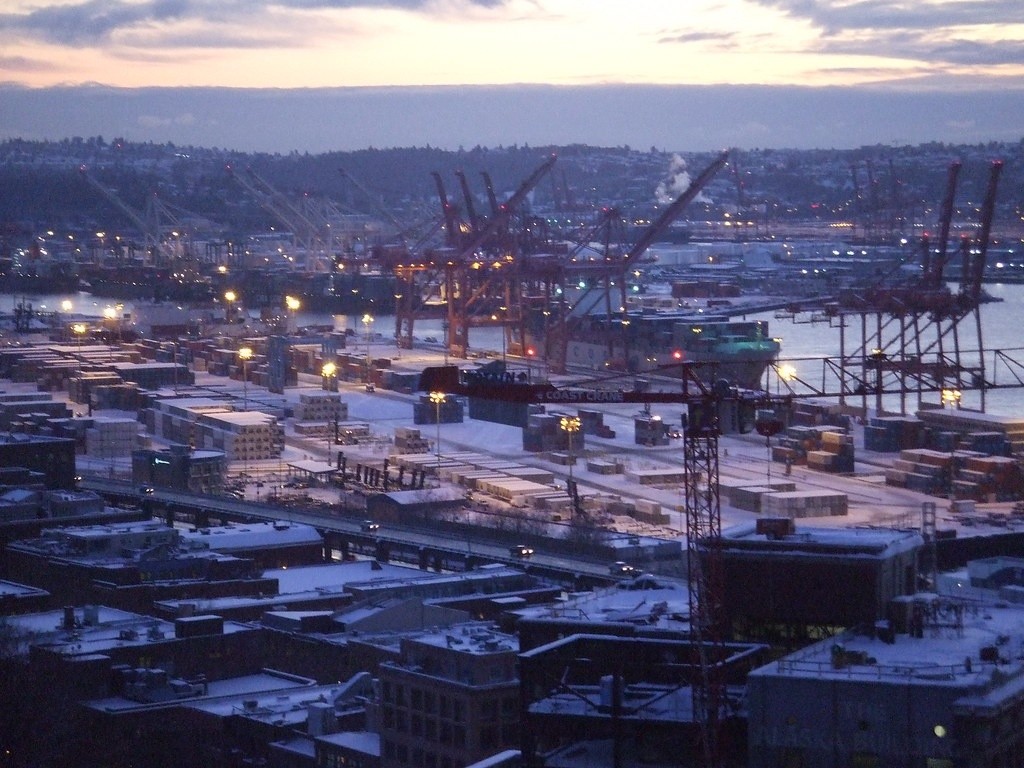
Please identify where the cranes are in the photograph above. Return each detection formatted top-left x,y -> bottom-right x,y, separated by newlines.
387,152 -> 729,374
825,157 -> 1003,415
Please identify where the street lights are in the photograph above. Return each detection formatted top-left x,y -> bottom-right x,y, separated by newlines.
428,390 -> 447,488
320,360 -> 338,467
285,296 -> 302,343
362,313 -> 374,384
557,415 -> 581,519
225,291 -> 237,333
237,348 -> 252,412
74,324 -> 86,384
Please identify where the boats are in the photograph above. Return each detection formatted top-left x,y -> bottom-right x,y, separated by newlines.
502,262 -> 782,389
